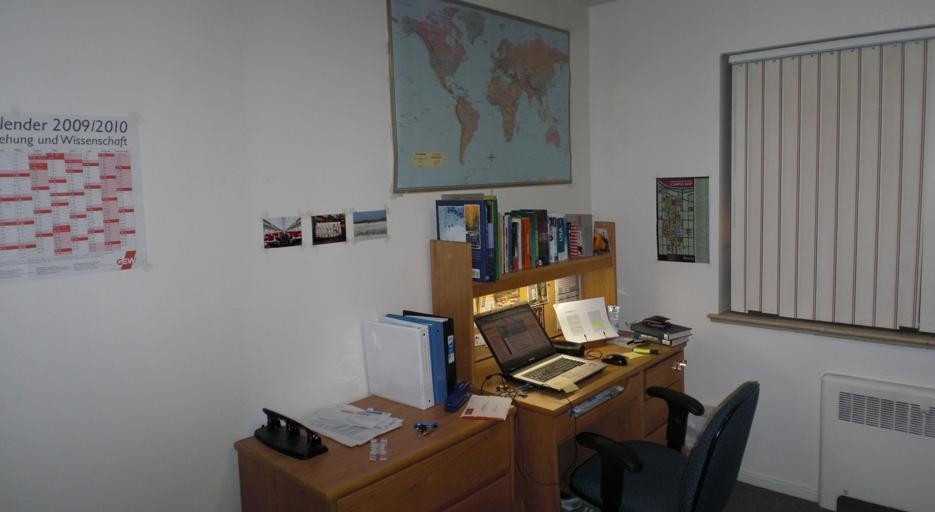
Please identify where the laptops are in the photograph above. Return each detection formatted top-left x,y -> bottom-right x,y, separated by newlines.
474,302 -> 608,392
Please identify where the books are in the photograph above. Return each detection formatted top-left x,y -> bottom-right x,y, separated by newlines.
459,394 -> 512,421
435,193 -> 595,283
634,332 -> 690,348
630,322 -> 694,340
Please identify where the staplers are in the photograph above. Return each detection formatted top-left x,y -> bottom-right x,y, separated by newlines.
446,381 -> 472,412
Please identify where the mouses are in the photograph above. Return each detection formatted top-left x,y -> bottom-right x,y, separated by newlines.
601,353 -> 627,366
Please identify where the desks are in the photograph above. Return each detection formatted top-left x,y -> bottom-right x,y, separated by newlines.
474,330 -> 685,507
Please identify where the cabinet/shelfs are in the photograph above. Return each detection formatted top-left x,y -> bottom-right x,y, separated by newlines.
430,223 -> 618,377
234,393 -> 515,511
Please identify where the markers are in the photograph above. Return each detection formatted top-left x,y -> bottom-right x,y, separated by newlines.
633,349 -> 660,353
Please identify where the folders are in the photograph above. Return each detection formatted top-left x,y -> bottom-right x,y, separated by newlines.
358,309 -> 458,410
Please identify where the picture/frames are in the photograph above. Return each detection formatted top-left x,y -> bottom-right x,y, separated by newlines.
386,2 -> 572,194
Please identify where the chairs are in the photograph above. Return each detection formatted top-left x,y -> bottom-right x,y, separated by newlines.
569,386 -> 760,511
